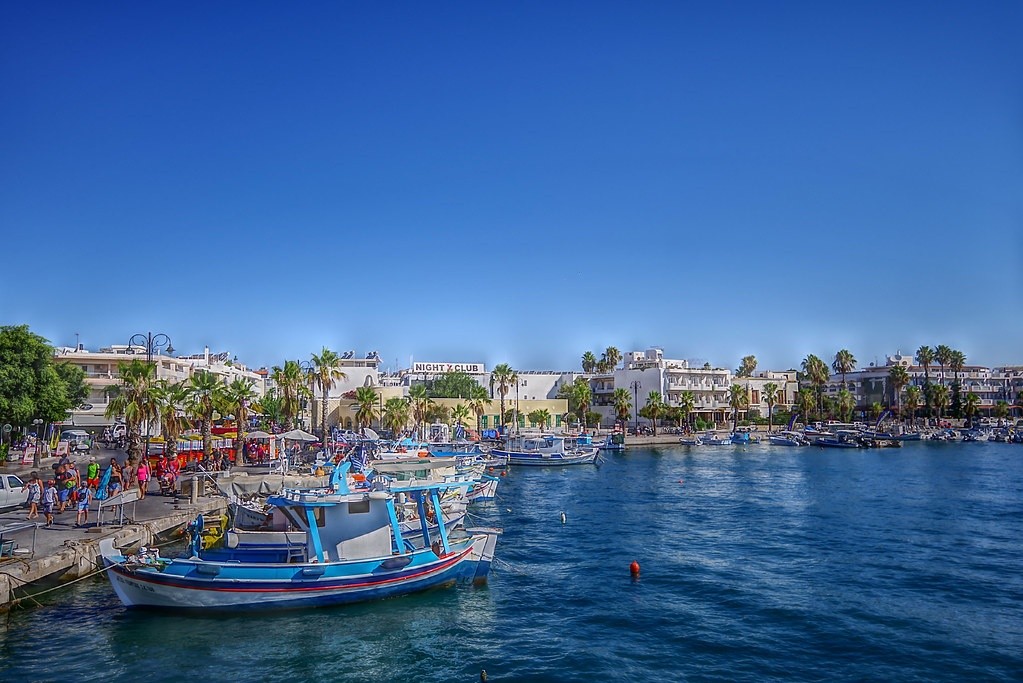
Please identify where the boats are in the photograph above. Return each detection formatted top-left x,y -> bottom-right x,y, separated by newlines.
603,432 -> 626,449
678,410 -> 1022,449
100,433 -> 600,612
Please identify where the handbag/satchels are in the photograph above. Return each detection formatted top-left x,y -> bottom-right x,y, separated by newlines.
80,489 -> 88,502
65,473 -> 76,489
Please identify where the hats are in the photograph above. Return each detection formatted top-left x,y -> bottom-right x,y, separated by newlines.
81,481 -> 88,487
160,455 -> 163,459
89,457 -> 96,462
172,453 -> 177,457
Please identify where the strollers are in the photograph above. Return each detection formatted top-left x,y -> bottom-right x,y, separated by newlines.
158,471 -> 177,497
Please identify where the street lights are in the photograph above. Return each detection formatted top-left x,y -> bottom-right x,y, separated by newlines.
510,375 -> 524,431
126,332 -> 176,461
629,381 -> 642,437
33,419 -> 44,461
287,359 -> 314,430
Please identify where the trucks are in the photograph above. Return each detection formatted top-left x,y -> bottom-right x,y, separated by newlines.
60,429 -> 90,454
110,423 -> 154,443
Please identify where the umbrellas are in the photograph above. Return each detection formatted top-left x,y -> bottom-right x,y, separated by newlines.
276,429 -> 319,441
246,431 -> 269,439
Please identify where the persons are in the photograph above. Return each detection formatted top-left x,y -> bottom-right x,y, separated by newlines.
13,432 -> 44,465
636,423 -> 654,437
153,451 -> 181,492
211,439 -> 313,471
85,455 -> 151,499
20,452 -> 92,528
89,427 -> 123,450
662,422 -> 691,436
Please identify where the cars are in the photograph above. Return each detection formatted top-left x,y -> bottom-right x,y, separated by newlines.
0,474 -> 31,508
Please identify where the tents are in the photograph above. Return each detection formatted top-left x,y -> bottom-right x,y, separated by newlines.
189,434 -> 222,451
144,435 -> 187,450
223,431 -> 249,447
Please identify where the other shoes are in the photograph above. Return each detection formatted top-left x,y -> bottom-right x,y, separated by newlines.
57,510 -> 65,514
26,517 -> 30,520
32,514 -> 39,520
51,516 -> 54,525
44,524 -> 51,528
